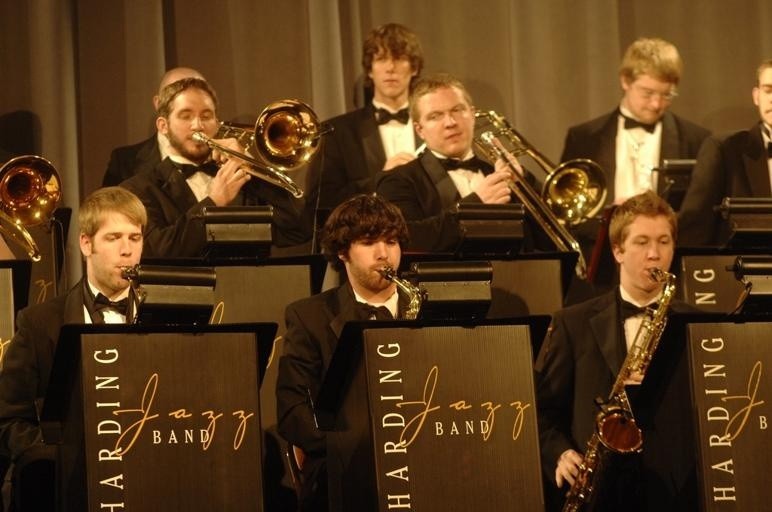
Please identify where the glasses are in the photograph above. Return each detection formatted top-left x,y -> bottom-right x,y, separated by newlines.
632,84 -> 679,100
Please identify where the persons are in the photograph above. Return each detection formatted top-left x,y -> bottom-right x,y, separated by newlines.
560,38 -> 721,245
0,185 -> 148,512
117,78 -> 314,259
721,61 -> 772,199
102,66 -> 206,187
274,194 -> 406,510
534,191 -> 691,512
376,73 -> 537,253
299,22 -> 428,239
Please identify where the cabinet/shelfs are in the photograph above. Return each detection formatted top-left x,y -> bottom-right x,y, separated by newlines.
682,253 -> 741,320
55,334 -> 265,512
489,259 -> 563,320
652,323 -> 770,510
215,265 -> 310,323
326,324 -> 545,510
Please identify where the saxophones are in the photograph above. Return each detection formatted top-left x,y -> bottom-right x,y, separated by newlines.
563,268 -> 676,512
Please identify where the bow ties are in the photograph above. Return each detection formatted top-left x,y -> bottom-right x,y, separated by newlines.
180,159 -> 216,180
625,116 -> 655,134
357,301 -> 393,319
440,154 -> 478,173
92,292 -> 129,317
622,301 -> 658,318
377,107 -> 409,125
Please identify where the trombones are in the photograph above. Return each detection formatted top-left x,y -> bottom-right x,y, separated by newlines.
193,97 -> 323,198
0,155 -> 62,262
471,108 -> 608,279
